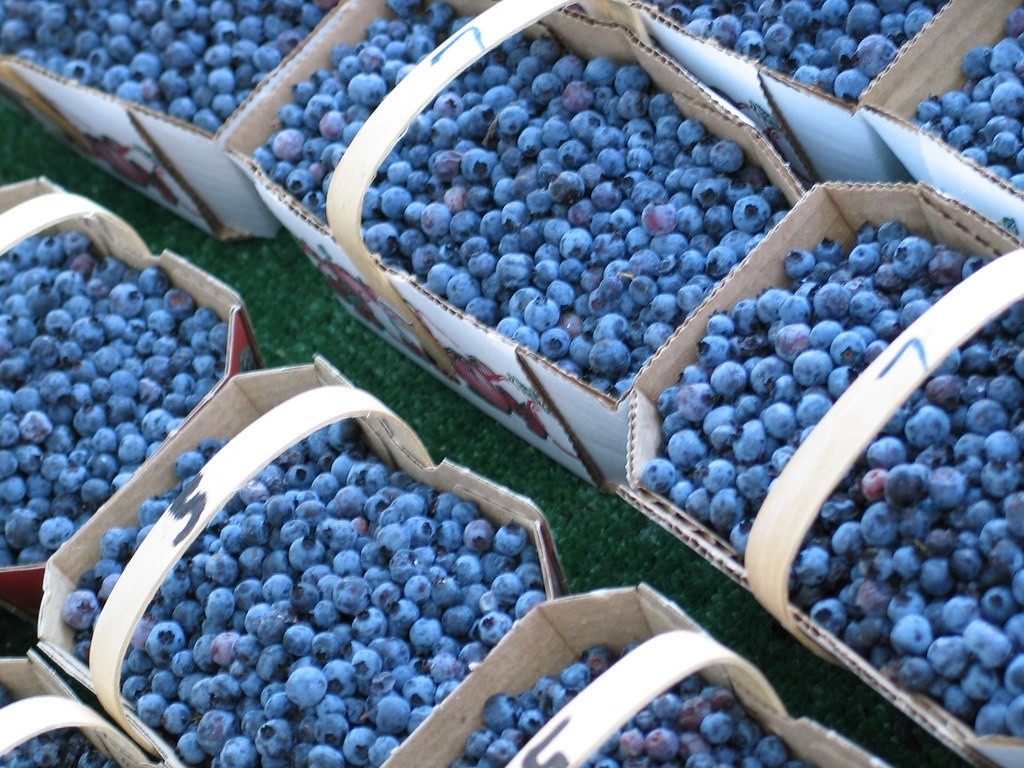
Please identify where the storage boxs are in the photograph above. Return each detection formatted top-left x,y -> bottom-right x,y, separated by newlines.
0,1 -> 1024,768
